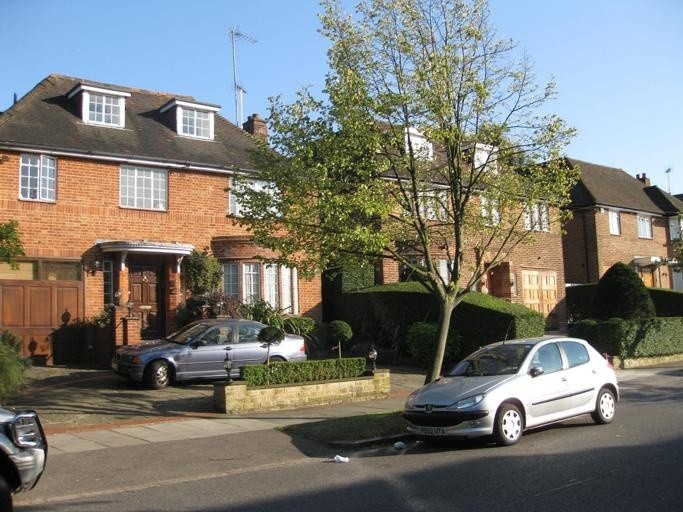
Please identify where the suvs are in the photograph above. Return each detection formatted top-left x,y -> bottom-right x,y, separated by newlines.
0,401 -> 48,512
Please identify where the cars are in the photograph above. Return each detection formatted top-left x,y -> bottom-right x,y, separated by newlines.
113,315 -> 309,390
398,332 -> 620,452
